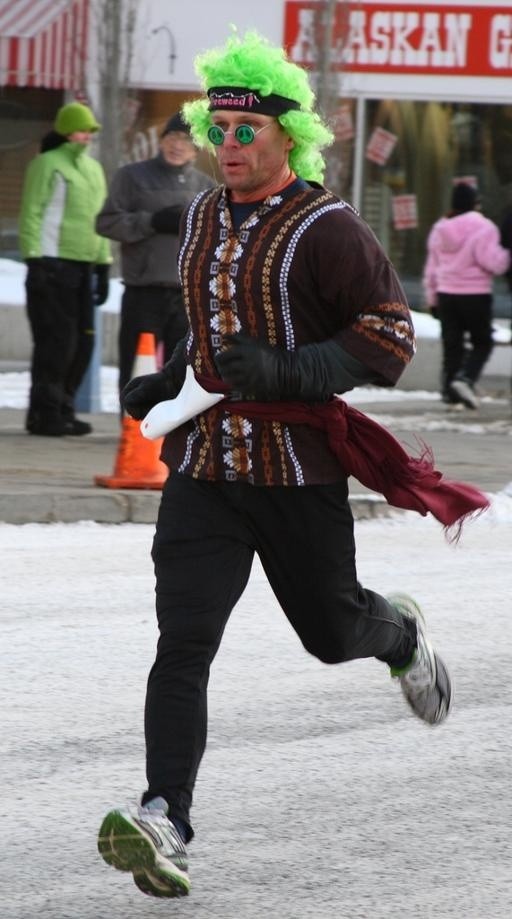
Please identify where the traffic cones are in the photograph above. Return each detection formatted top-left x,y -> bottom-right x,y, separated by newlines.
91,331 -> 171,491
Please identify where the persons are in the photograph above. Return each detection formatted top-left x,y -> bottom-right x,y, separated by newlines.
421,172 -> 511,411
17,100 -> 117,438
88,19 -> 461,899
95,109 -> 221,436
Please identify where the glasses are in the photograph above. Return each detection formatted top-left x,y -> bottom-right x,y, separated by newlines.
207,124 -> 271,145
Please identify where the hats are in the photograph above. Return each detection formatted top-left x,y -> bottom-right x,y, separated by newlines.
160,112 -> 191,139
54,102 -> 103,136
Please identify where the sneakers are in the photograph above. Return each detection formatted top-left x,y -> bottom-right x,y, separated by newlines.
97,795 -> 192,899
24,419 -> 95,436
440,379 -> 482,410
384,590 -> 456,728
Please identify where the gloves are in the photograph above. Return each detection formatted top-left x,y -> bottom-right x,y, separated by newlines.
213,335 -> 378,414
119,338 -> 187,421
